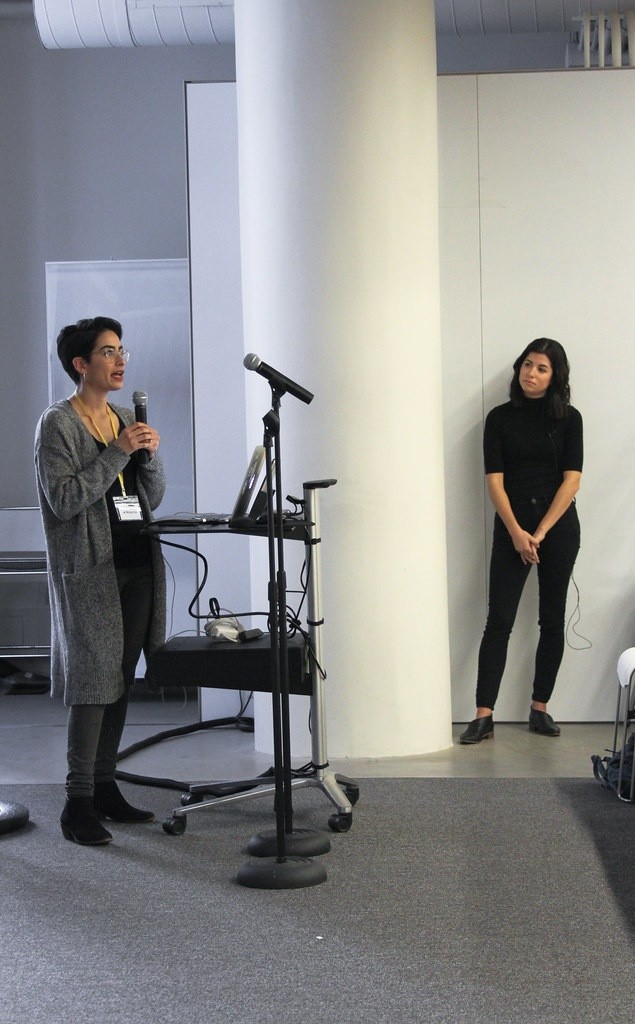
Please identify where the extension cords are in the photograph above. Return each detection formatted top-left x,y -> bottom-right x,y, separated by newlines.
209,620 -> 238,644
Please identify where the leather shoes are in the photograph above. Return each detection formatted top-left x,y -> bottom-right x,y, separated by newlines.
460,714 -> 495,743
528,704 -> 561,736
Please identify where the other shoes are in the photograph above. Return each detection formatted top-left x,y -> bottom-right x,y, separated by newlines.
61,797 -> 112,845
94,782 -> 155,823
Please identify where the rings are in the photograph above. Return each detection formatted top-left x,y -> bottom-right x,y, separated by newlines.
149,439 -> 152,443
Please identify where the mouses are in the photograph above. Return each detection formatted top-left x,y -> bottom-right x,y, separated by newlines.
255,512 -> 283,524
225,517 -> 256,528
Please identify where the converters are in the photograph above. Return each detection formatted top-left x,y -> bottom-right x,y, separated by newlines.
238,628 -> 266,643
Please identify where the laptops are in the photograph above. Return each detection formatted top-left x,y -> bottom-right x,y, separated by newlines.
148,445 -> 267,524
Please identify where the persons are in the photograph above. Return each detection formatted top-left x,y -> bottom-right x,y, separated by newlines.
33,315 -> 166,846
459,338 -> 583,744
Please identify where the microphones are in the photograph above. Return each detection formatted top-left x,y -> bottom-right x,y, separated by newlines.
242,352 -> 314,407
131,391 -> 149,464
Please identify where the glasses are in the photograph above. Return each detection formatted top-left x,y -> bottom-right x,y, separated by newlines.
88,349 -> 130,364
